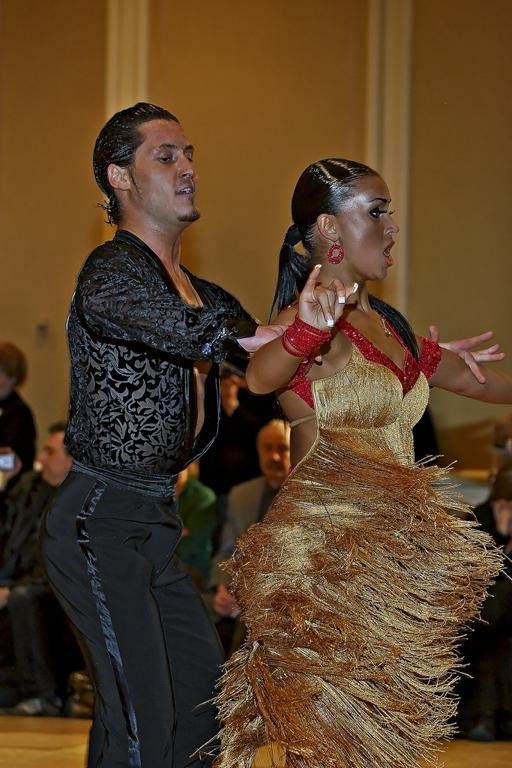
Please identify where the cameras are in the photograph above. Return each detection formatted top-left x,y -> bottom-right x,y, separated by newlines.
0,452 -> 15,472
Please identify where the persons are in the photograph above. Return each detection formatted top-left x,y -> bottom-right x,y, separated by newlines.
1,344 -> 511,742
36,104 -> 507,768
187,159 -> 512,768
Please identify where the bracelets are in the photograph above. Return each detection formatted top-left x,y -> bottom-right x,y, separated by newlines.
281,313 -> 332,357
